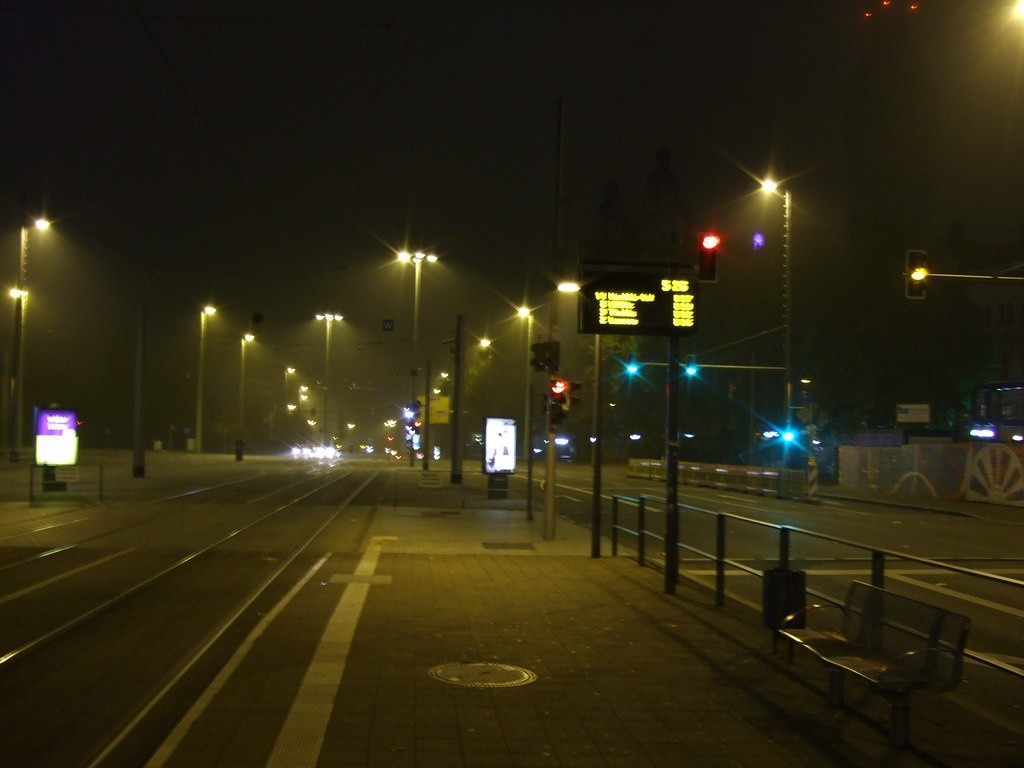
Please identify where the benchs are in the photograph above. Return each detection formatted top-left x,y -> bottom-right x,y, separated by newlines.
772,579 -> 972,749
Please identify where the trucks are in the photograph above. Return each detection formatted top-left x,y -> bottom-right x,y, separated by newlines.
952,382 -> 1024,444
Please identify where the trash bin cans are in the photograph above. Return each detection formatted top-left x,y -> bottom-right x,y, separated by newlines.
761,566 -> 807,664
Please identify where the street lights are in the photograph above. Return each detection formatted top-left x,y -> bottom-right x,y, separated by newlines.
760,176 -> 794,469
197,304 -> 217,454
283,366 -> 296,403
315,312 -> 344,448
13,217 -> 51,451
422,357 -> 450,471
516,304 -> 533,461
237,332 -> 256,435
556,276 -> 604,559
396,249 -> 438,467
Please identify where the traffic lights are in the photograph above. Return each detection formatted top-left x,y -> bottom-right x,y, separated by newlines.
697,231 -> 720,282
550,379 -> 582,406
905,249 -> 930,300
530,340 -> 560,375
